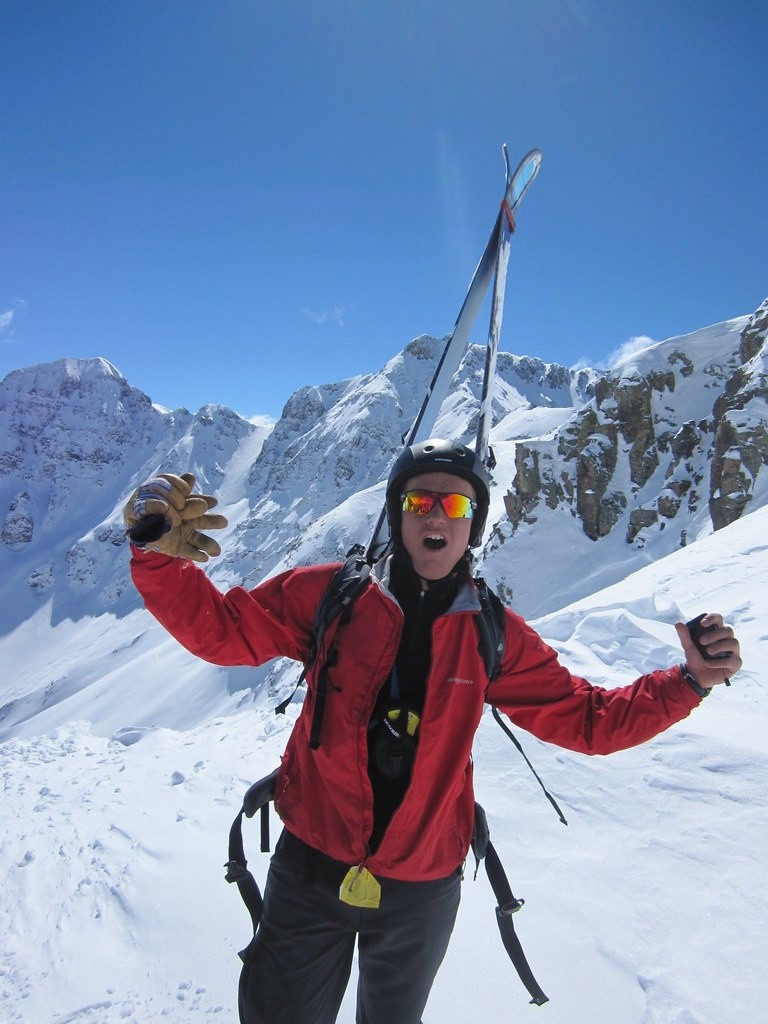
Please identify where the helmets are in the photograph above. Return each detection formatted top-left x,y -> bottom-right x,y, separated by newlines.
386,438 -> 490,547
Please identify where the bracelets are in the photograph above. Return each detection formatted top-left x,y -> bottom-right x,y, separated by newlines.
679,660 -> 712,699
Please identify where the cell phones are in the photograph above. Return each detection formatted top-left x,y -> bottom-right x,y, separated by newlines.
685,613 -> 732,660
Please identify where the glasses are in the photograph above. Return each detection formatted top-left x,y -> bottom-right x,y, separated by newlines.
400,489 -> 479,519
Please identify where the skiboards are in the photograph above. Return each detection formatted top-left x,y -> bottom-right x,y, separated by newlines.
370,140 -> 545,579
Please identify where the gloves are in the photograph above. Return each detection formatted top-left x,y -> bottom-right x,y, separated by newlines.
123,473 -> 228,563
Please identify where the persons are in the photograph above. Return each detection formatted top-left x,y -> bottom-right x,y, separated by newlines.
124,438 -> 743,1024
